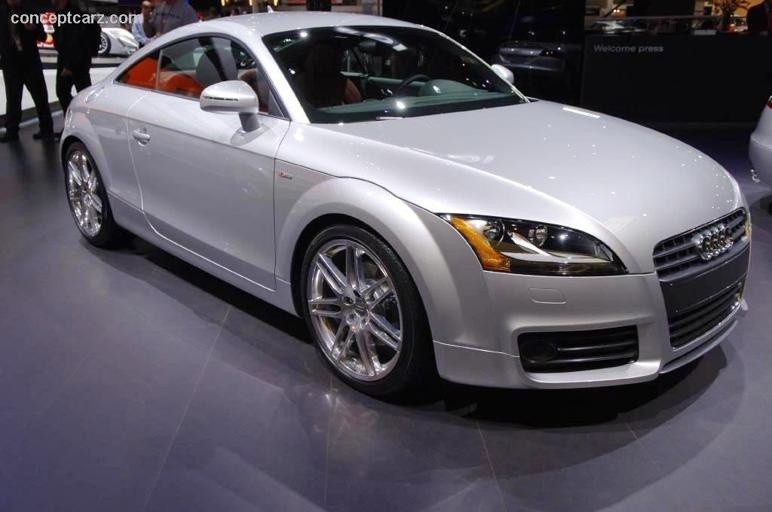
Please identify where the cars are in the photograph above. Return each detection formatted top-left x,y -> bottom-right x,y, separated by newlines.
747,93 -> 772,192
34,10 -> 140,56
58,11 -> 751,404
495,6 -> 612,93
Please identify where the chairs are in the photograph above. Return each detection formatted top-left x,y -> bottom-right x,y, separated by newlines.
187,42 -> 363,112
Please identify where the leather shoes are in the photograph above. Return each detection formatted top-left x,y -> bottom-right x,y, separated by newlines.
0,132 -> 19,143
34,127 -> 53,139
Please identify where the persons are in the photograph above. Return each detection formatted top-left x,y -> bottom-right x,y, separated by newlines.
0,0 -> 54,148
154,1 -> 196,69
131,1 -> 159,60
52,0 -> 101,142
229,8 -> 242,69
